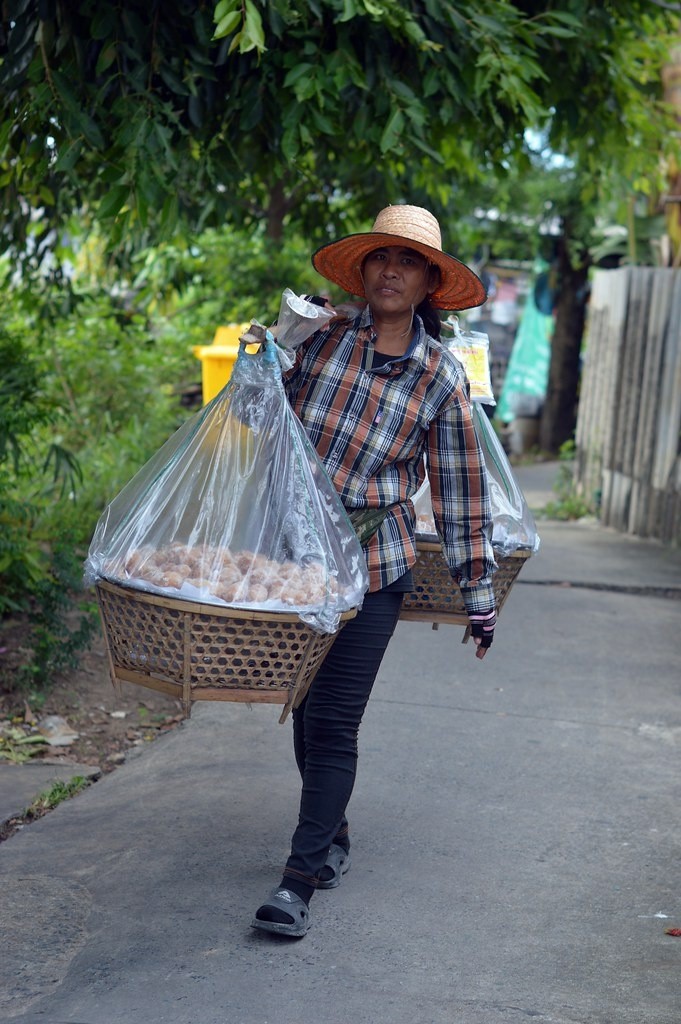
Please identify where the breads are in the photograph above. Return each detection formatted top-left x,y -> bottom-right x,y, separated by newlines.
121,540 -> 339,605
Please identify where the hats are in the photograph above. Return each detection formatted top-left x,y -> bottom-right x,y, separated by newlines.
311,205 -> 488,311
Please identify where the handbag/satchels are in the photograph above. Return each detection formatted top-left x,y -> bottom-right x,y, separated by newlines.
438,320 -> 496,405
273,499 -> 402,560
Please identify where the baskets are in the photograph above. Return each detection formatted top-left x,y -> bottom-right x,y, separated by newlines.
395,533 -> 533,645
96,578 -> 358,725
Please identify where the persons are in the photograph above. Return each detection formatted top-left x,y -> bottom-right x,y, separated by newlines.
229,202 -> 499,936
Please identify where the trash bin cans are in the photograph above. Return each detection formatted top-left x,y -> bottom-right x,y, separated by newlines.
191,322 -> 260,451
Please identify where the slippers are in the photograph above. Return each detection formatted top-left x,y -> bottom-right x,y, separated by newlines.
315,842 -> 350,889
249,887 -> 312,938
